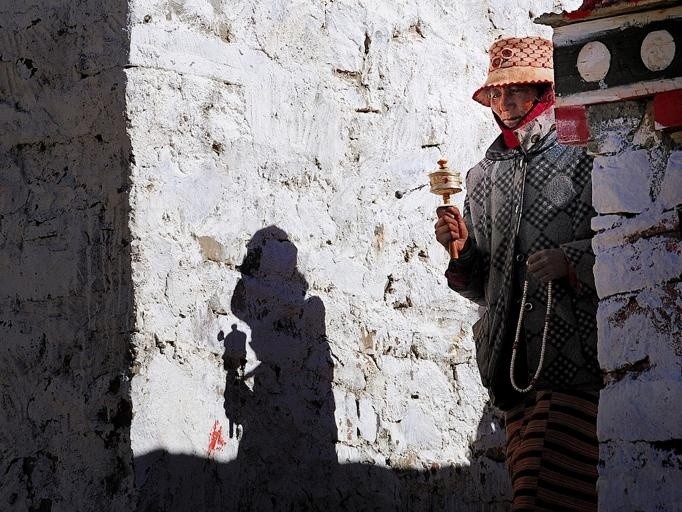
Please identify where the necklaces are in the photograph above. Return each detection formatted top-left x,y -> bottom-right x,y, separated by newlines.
510,260 -> 552,394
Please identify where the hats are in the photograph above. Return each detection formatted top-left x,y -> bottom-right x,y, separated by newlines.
471,36 -> 554,108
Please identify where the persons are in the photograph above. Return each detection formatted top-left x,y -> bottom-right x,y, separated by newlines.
435,35 -> 599,511
